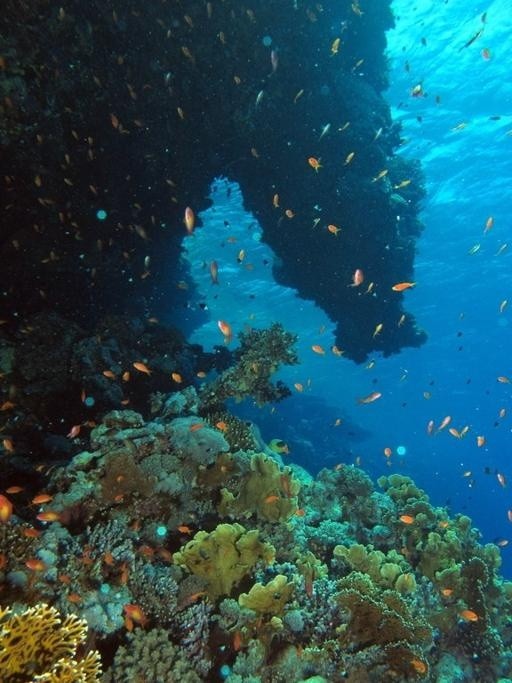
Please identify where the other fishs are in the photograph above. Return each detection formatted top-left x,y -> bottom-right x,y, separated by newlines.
0,0 -> 509,683
